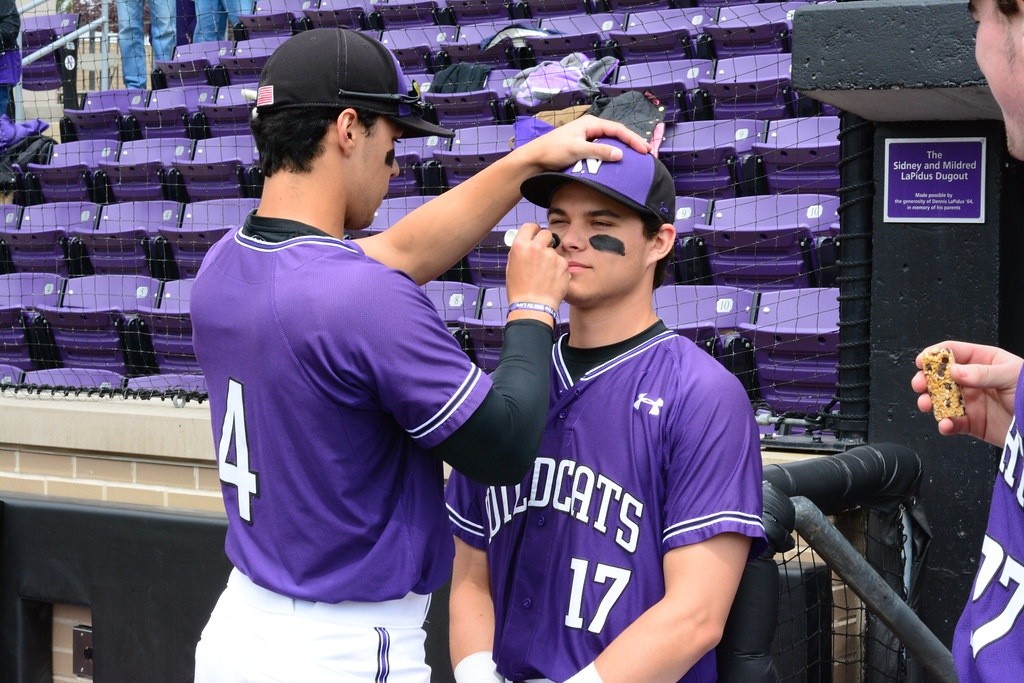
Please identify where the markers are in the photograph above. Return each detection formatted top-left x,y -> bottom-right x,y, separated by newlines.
503,229 -> 562,250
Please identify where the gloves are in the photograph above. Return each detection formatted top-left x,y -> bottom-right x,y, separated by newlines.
561,660 -> 604,683
454,652 -> 505,683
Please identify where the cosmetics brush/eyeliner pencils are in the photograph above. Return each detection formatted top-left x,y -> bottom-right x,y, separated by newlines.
504,229 -> 557,248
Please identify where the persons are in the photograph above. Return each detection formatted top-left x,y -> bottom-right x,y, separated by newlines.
192,26 -> 651,683
194,0 -> 255,43
910,0 -> 1024,683
0,0 -> 23,117
115,0 -> 177,90
449,136 -> 769,683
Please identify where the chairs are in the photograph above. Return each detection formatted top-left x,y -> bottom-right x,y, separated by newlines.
0,0 -> 852,452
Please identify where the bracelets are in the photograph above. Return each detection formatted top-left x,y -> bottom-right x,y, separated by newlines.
506,302 -> 557,329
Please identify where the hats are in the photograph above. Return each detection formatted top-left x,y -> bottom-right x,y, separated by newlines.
251,25 -> 456,139
520,137 -> 675,225
